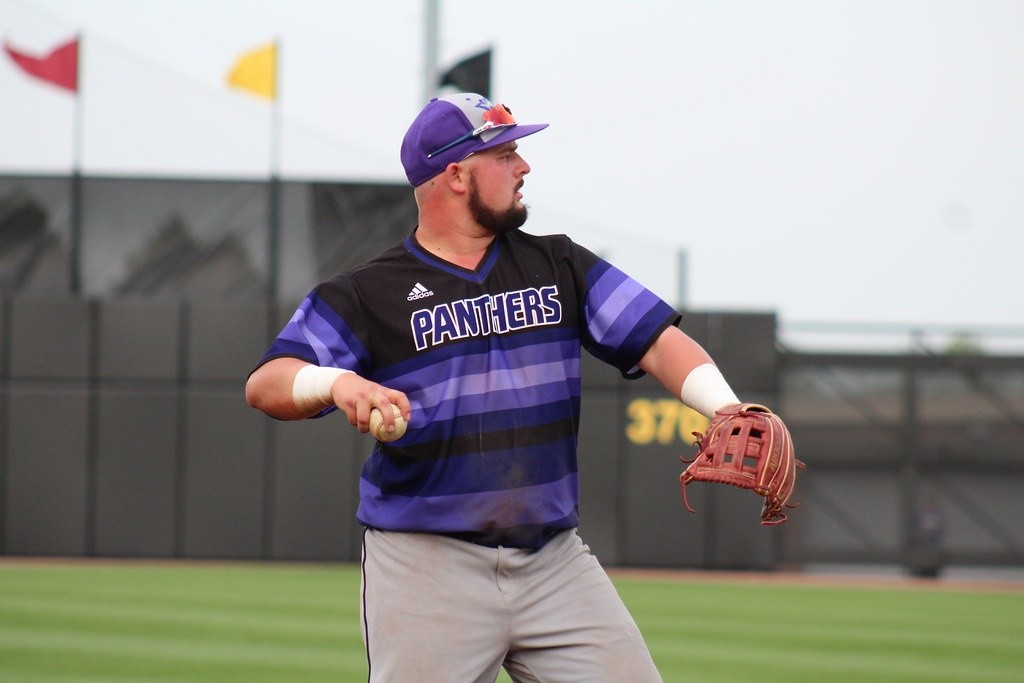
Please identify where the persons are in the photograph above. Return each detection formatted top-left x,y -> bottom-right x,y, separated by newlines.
241,93 -> 742,683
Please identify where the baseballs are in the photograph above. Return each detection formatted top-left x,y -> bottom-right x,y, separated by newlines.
368,404 -> 409,443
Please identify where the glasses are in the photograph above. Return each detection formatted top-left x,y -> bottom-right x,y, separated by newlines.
427,103 -> 517,159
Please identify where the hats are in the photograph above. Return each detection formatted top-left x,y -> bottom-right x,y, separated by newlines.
401,93 -> 549,187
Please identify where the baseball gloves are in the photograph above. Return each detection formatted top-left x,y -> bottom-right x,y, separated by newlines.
677,400 -> 798,523
